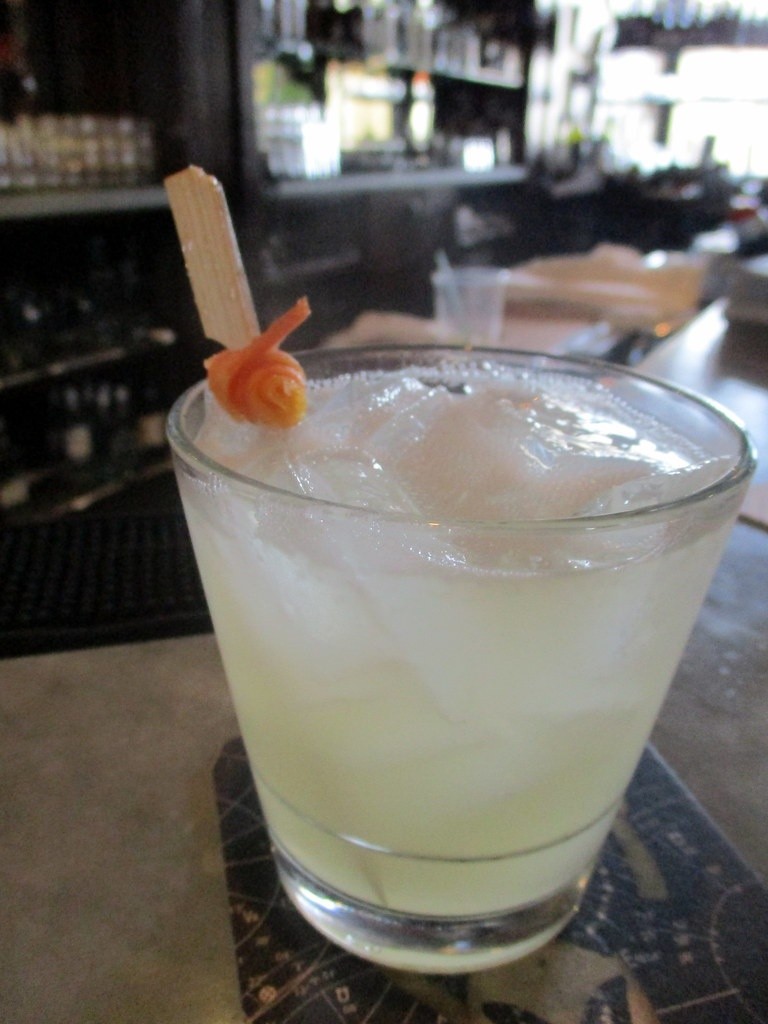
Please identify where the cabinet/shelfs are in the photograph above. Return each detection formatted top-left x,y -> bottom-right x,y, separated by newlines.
0,0 -> 533,526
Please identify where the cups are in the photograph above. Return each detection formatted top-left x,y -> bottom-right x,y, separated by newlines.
429,267 -> 507,346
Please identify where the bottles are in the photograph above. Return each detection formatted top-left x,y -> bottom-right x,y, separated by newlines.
167,345 -> 756,978
0,113 -> 156,192
240,0 -> 768,182
728,194 -> 758,248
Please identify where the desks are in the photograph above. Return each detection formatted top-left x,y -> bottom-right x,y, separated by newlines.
0,299 -> 767,1024
